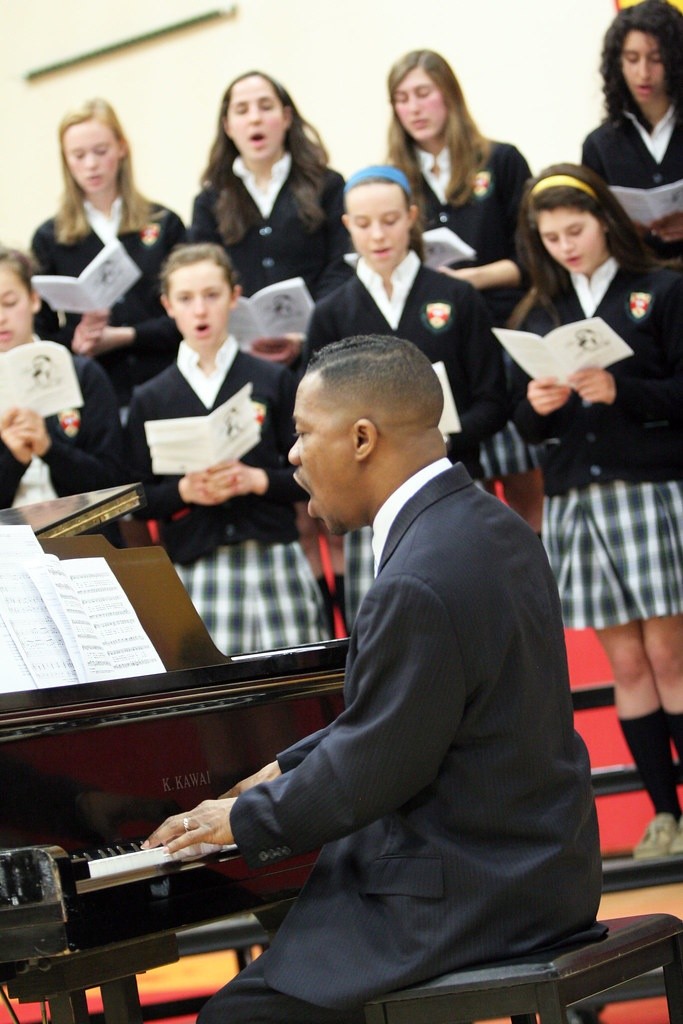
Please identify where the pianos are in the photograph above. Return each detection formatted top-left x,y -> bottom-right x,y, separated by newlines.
0,484 -> 349,1024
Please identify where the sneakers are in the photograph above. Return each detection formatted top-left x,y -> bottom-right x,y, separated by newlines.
633,813 -> 683,860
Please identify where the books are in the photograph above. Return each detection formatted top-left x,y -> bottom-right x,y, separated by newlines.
0,549 -> 167,693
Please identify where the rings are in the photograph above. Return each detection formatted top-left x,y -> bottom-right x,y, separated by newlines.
183,818 -> 189,832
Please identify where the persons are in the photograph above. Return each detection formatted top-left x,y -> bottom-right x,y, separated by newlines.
0,0 -> 683,862
137,333 -> 605,1023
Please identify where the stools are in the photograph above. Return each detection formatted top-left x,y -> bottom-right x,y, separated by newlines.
365,914 -> 683,1024
